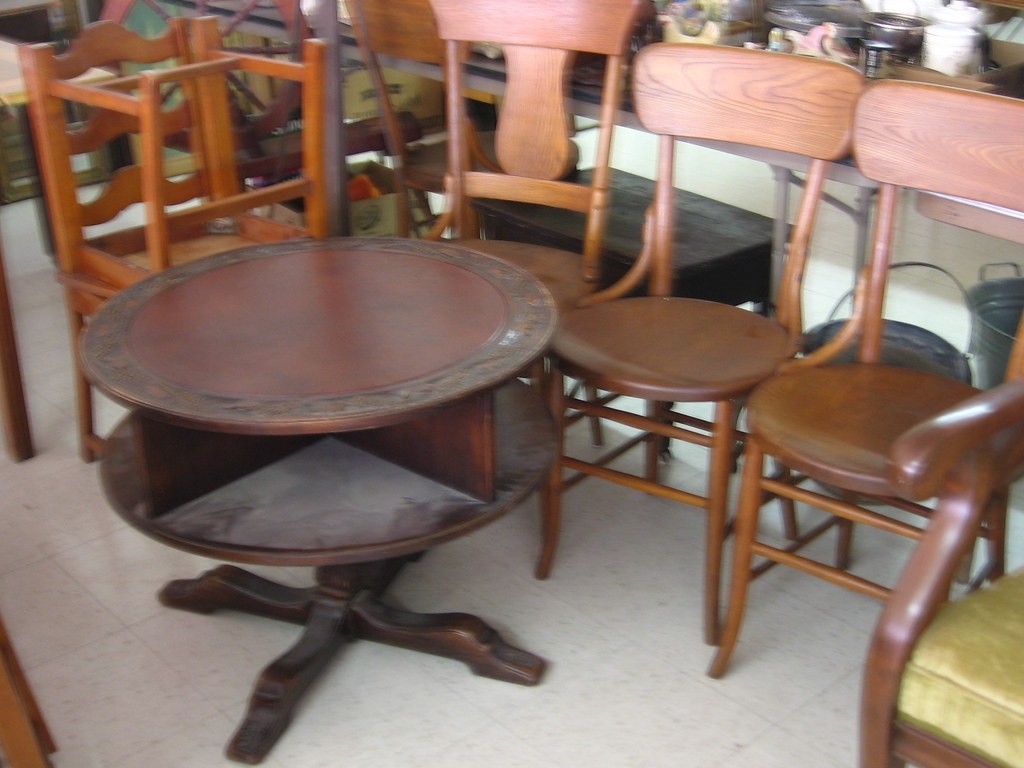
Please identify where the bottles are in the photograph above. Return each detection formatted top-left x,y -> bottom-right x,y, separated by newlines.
933,0 -> 983,27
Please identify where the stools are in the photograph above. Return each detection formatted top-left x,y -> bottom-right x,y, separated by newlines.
24,16 -> 325,461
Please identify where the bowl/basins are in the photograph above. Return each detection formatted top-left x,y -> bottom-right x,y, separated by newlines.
920,21 -> 981,80
862,13 -> 929,62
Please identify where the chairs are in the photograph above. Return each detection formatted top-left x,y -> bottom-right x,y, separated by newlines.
342,0 -> 1024,768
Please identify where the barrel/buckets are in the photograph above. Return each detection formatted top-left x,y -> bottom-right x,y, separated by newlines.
964,258 -> 1024,398
796,261 -> 977,504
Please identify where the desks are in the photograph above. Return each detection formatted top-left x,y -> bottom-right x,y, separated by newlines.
166,0 -> 1024,340
470,166 -> 798,473
79,238 -> 558,764
